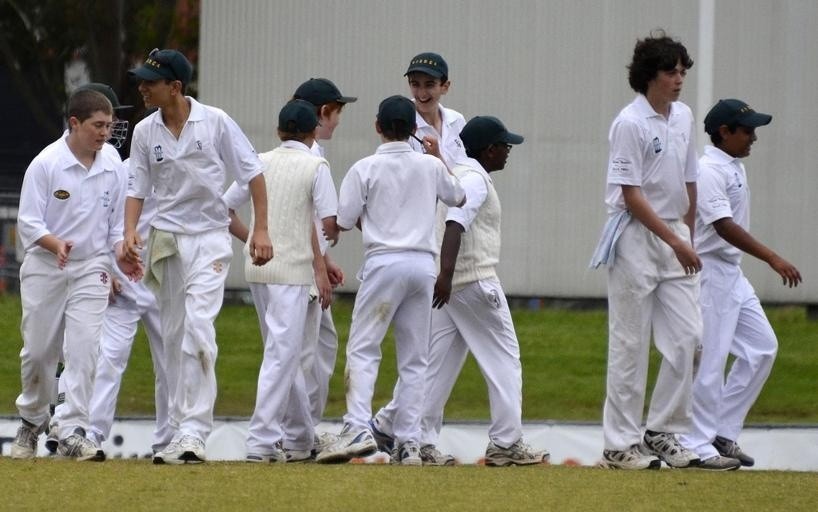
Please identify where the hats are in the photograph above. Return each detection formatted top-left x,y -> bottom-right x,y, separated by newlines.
278,78 -> 357,132
75,82 -> 134,110
704,99 -> 771,135
126,49 -> 192,84
375,95 -> 415,124
459,116 -> 522,150
403,53 -> 447,79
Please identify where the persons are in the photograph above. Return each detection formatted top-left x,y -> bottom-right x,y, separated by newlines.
368,52 -> 467,457
586,30 -> 704,471
316,95 -> 467,466
391,116 -> 550,467
122,48 -> 273,465
12,84 -> 173,463
274,78 -> 358,450
695,98 -> 804,474
222,101 -> 340,465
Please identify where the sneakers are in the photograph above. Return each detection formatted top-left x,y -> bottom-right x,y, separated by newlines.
152,435 -> 206,465
245,423 -> 455,468
9,419 -> 105,462
599,431 -> 753,472
484,438 -> 549,467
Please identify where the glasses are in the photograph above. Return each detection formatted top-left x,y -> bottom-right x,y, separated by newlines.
148,47 -> 179,80
